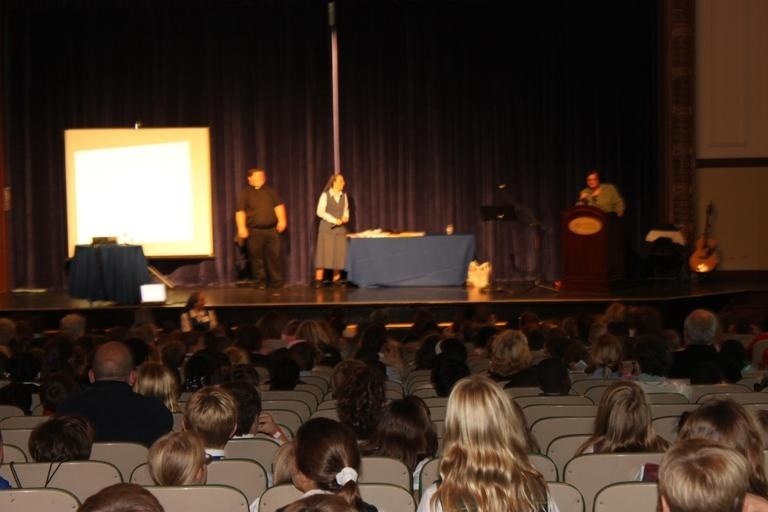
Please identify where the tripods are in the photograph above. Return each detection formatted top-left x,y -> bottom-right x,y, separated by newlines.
502,192 -> 559,294
480,220 -> 515,294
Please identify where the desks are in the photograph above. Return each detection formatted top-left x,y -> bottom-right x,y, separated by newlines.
343,231 -> 478,290
66,244 -> 152,305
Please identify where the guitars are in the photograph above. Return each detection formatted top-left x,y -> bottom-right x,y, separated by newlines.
688,201 -> 720,273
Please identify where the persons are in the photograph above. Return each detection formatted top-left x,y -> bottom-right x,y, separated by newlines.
575,169 -> 625,219
232,164 -> 287,290
309,172 -> 350,290
671,215 -> 705,249
180,291 -> 218,333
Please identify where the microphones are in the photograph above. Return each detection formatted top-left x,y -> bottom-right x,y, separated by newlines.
496,184 -> 505,190
581,193 -> 589,199
591,191 -> 599,197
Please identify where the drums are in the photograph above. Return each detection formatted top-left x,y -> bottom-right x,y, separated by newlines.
568,203 -> 604,235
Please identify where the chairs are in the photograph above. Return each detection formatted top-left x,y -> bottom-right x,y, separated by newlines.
0,352 -> 768,512
